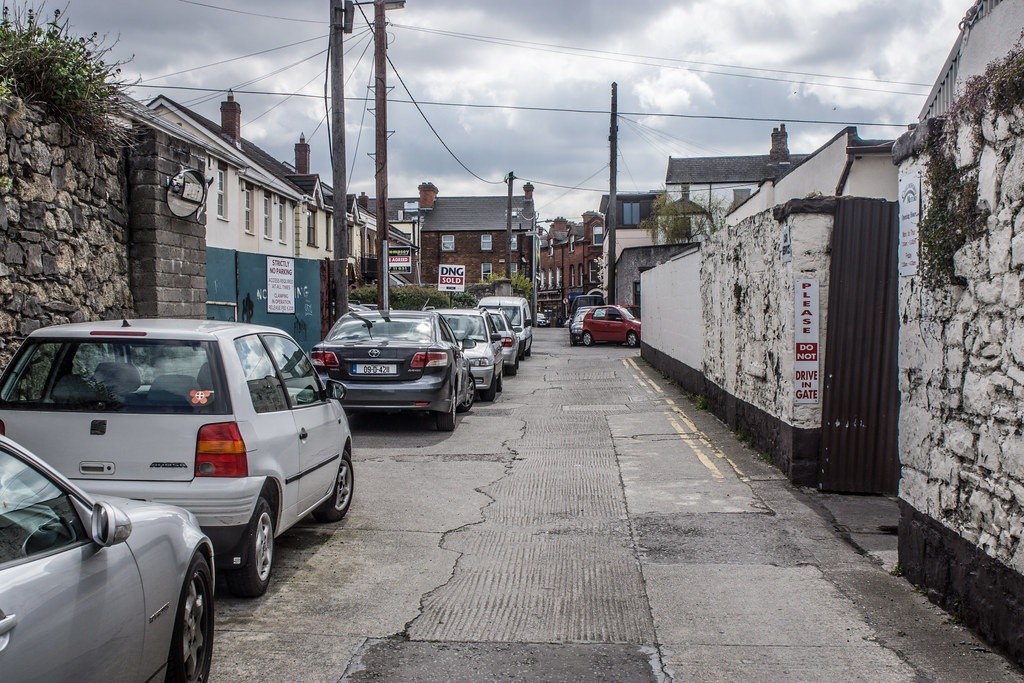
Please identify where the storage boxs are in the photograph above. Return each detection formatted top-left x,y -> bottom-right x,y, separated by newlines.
468,335 -> 485,340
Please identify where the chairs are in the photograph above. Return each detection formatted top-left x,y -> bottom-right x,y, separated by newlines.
407,322 -> 431,342
448,317 -> 460,330
343,320 -> 376,335
197,362 -> 217,401
146,374 -> 201,406
461,318 -> 480,339
52,373 -> 107,404
94,361 -> 141,396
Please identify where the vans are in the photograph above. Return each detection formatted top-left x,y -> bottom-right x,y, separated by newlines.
475,296 -> 533,361
567,295 -> 605,332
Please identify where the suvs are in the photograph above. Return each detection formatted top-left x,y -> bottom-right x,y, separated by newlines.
452,307 -> 522,375
421,306 -> 503,402
0,318 -> 354,599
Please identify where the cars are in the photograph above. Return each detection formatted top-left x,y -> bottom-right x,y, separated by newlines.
537,317 -> 550,327
569,306 -> 597,345
311,310 -> 476,431
582,305 -> 641,347
172,174 -> 184,186
1,432 -> 216,683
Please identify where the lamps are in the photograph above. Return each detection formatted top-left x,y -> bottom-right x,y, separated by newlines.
498,258 -> 505,264
